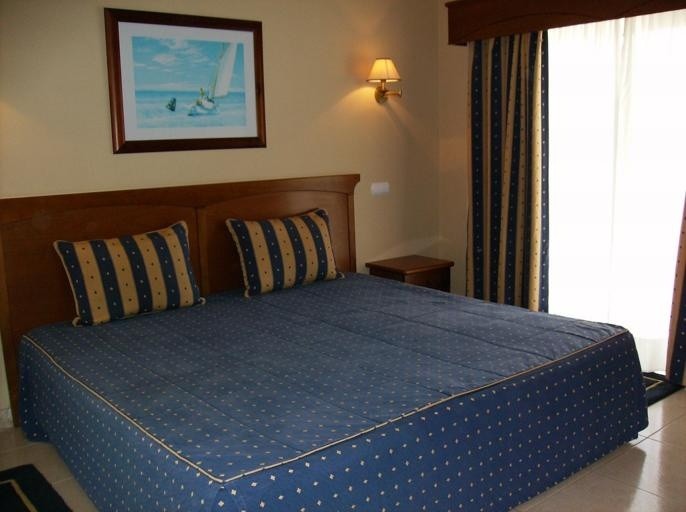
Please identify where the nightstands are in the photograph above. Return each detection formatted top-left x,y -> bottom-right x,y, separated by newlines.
365,255 -> 454,293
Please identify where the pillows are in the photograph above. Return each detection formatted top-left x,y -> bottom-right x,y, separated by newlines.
51,207 -> 345,327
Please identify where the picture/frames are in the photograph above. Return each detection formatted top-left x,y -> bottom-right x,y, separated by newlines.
103,6 -> 267,154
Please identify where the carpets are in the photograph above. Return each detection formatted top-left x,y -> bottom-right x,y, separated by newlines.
1,464 -> 74,511
641,373 -> 686,407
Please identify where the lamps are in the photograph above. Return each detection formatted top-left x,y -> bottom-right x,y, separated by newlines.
365,58 -> 404,104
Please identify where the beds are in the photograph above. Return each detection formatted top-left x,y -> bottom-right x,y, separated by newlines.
1,173 -> 637,511
